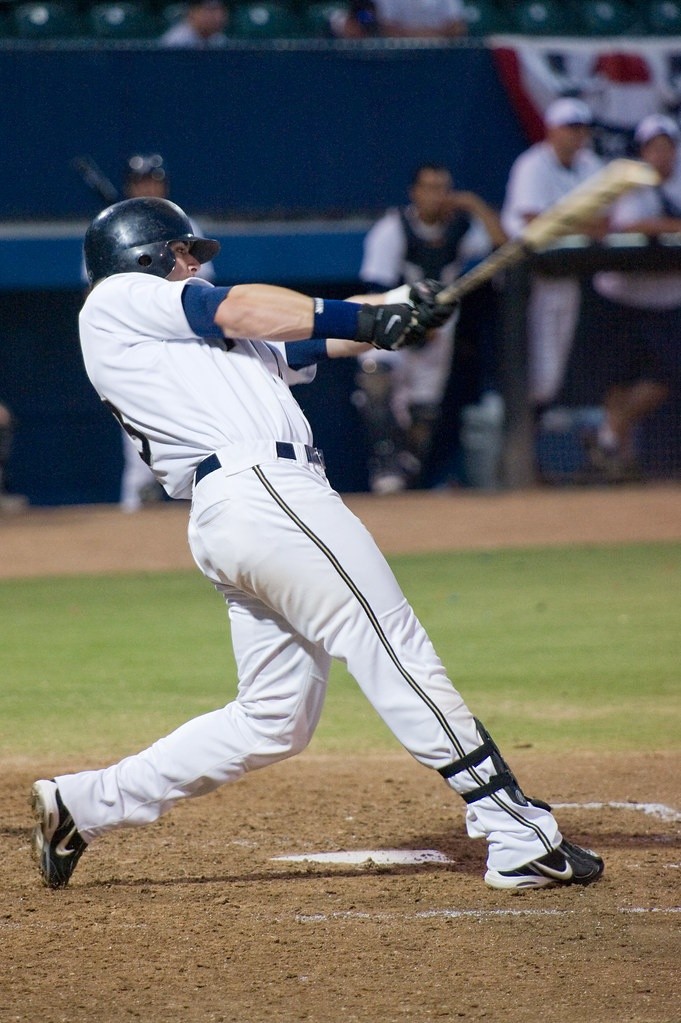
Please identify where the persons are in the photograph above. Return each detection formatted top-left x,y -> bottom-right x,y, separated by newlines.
31,198 -> 603,889
83,160 -> 218,505
353,165 -> 507,490
328,0 -> 468,38
502,97 -> 680,489
166,0 -> 238,41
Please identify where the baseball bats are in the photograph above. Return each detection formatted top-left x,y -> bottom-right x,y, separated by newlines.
434,159 -> 658,306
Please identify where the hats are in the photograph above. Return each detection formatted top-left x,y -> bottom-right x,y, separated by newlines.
635,113 -> 679,145
543,96 -> 592,130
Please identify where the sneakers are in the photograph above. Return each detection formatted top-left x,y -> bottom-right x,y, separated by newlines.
485,838 -> 604,890
31,779 -> 89,889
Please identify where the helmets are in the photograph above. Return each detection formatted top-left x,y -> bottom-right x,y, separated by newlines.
84,197 -> 221,291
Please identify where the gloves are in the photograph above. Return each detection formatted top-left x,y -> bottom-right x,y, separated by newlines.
385,279 -> 458,328
357,301 -> 428,350
